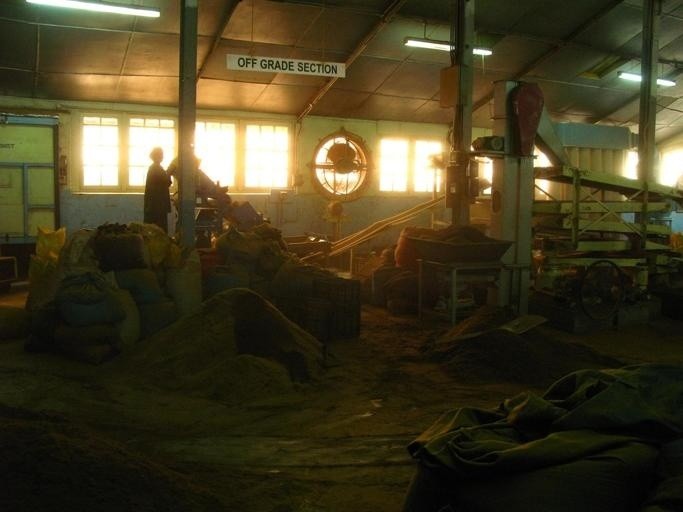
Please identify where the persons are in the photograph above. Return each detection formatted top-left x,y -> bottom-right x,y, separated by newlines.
143,147 -> 176,236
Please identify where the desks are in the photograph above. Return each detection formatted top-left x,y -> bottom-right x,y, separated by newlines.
417,258 -> 525,329
